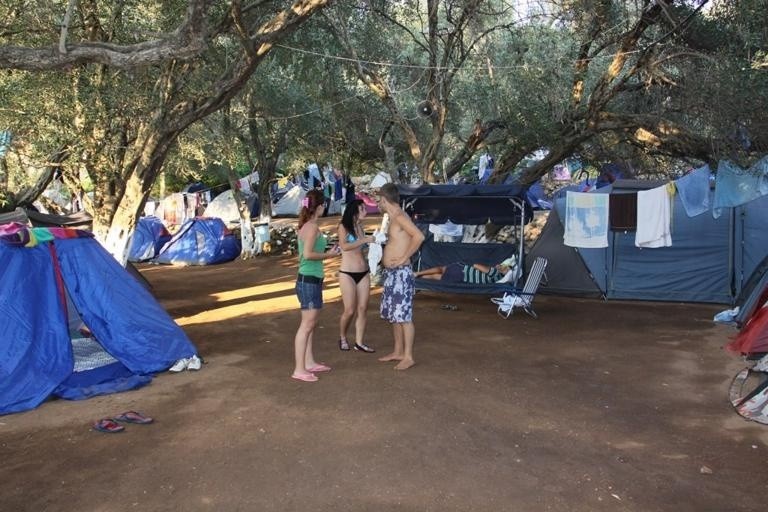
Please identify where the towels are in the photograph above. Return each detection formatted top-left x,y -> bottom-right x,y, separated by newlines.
562,153 -> 768,256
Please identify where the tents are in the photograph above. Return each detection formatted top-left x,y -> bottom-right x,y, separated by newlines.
202,188 -> 260,229
153,193 -> 205,236
734,253 -> 768,330
127,215 -> 172,263
523,179 -> 768,304
154,216 -> 241,266
726,289 -> 768,355
0,221 -> 204,417
399,184 -> 533,296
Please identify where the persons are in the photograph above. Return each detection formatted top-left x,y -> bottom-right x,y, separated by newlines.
291,189 -> 339,382
414,261 -> 513,285
378,183 -> 425,371
337,199 -> 376,354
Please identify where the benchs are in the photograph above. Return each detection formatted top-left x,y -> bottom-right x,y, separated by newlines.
405,238 -> 525,298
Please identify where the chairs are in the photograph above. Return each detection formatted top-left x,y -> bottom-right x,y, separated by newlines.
488,256 -> 549,320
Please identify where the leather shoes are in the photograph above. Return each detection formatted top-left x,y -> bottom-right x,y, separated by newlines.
115,410 -> 153,424
91,418 -> 125,433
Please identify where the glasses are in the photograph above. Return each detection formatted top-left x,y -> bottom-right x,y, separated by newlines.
322,201 -> 327,209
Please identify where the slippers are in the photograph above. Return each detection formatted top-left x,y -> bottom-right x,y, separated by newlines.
292,372 -> 318,382
305,364 -> 330,372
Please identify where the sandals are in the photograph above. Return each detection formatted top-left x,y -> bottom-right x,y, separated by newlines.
340,337 -> 350,350
354,344 -> 375,353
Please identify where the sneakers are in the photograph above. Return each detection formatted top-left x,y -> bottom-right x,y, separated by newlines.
187,355 -> 202,371
168,357 -> 192,373
441,304 -> 458,311
